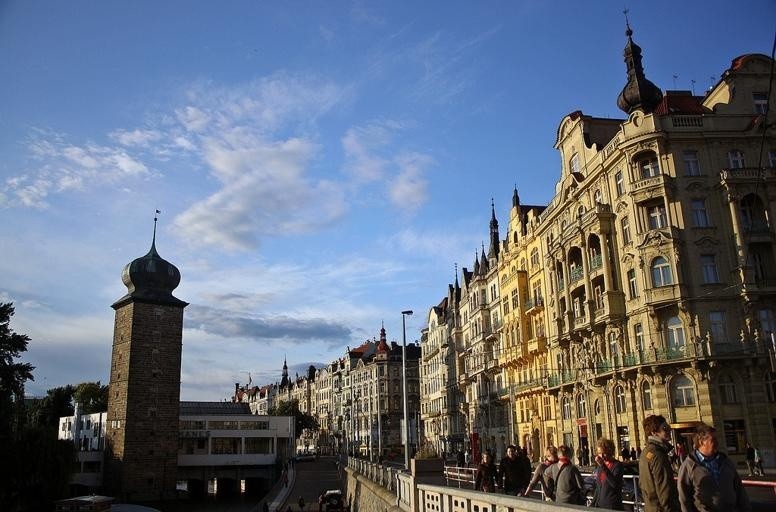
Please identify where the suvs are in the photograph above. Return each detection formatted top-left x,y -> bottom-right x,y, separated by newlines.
325,489 -> 343,511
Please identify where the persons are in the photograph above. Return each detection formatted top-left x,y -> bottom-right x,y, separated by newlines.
476,412 -> 765,512
284,473 -> 289,488
378,452 -> 384,463
465,449 -> 472,468
457,448 -> 464,472
286,506 -> 292,512
263,502 -> 268,511
318,493 -> 325,512
299,496 -> 305,512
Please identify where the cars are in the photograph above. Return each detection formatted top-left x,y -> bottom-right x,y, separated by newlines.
296,452 -> 314,462
582,464 -> 645,512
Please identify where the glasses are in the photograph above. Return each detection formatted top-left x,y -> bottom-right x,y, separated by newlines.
662,425 -> 671,432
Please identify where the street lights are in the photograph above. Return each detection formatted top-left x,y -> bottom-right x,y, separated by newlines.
401,308 -> 414,473
